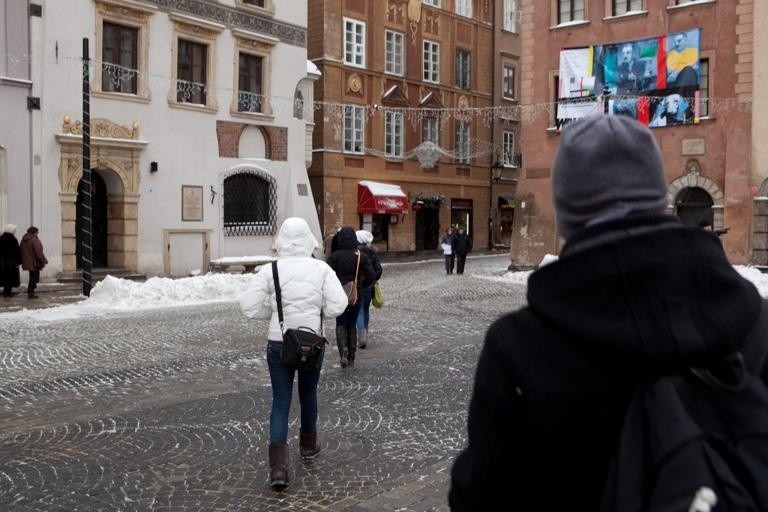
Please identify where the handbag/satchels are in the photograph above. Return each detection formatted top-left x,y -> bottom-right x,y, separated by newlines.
32,256 -> 45,269
281,326 -> 326,371
341,280 -> 359,306
372,283 -> 383,309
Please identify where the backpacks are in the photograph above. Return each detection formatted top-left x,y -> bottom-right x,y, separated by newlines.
613,298 -> 768,512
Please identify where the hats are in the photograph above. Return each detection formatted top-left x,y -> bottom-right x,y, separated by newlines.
4,224 -> 16,233
551,112 -> 668,236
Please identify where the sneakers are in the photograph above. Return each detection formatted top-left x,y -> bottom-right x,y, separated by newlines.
29,295 -> 39,298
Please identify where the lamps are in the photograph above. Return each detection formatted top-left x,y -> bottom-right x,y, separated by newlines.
491,144 -> 522,184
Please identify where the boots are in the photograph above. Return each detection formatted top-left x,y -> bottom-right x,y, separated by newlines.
298,429 -> 321,460
336,323 -> 369,367
269,442 -> 290,487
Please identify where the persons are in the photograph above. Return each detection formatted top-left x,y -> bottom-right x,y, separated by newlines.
699,219 -> 730,238
0,224 -> 20,298
447,114 -> 766,511
323,225 -> 383,369
555,29 -> 700,130
441,228 -> 457,275
20,227 -> 49,298
500,214 -> 513,237
240,217 -> 349,489
452,226 -> 473,274
